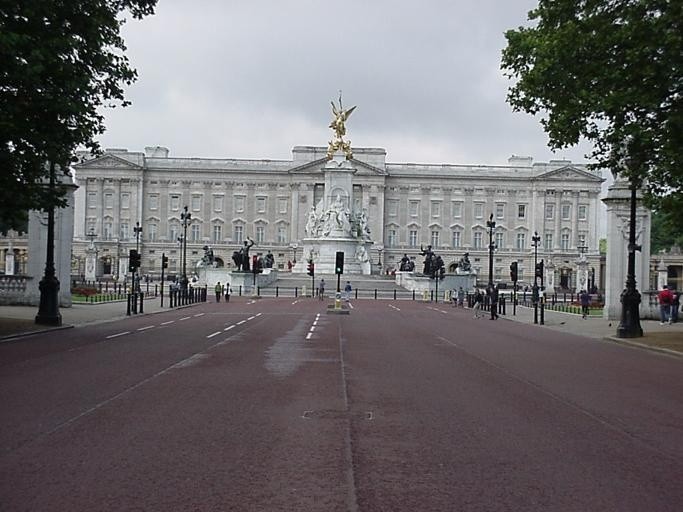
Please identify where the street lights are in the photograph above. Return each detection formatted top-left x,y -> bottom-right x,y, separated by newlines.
178,206 -> 191,275
486,212 -> 496,283
133,222 -> 142,248
532,231 -> 541,285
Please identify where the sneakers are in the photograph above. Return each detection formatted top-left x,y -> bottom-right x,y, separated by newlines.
659,321 -> 671,326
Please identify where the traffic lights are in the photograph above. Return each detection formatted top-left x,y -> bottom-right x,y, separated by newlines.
253,255 -> 263,274
129,250 -> 142,273
336,252 -> 344,274
430,261 -> 445,280
511,262 -> 518,281
537,260 -> 543,277
162,254 -> 169,268
308,260 -> 314,276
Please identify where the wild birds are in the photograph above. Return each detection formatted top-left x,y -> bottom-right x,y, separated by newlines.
560,321 -> 565,325
609,323 -> 612,327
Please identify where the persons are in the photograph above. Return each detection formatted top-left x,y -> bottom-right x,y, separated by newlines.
471,288 -> 482,318
172,280 -> 175,288
224,282 -> 231,302
344,281 -> 351,302
580,289 -> 587,318
174,280 -> 178,288
489,287 -> 499,320
214,282 -> 221,302
397,253 -> 409,271
417,243 -> 435,273
452,289 -> 457,307
318,278 -> 324,302
658,285 -> 673,325
458,253 -> 470,271
303,194 -> 371,239
200,246 -> 212,265
669,285 -> 679,322
238,236 -> 254,270
265,250 -> 273,267
457,286 -> 464,308
134,273 -> 141,292
